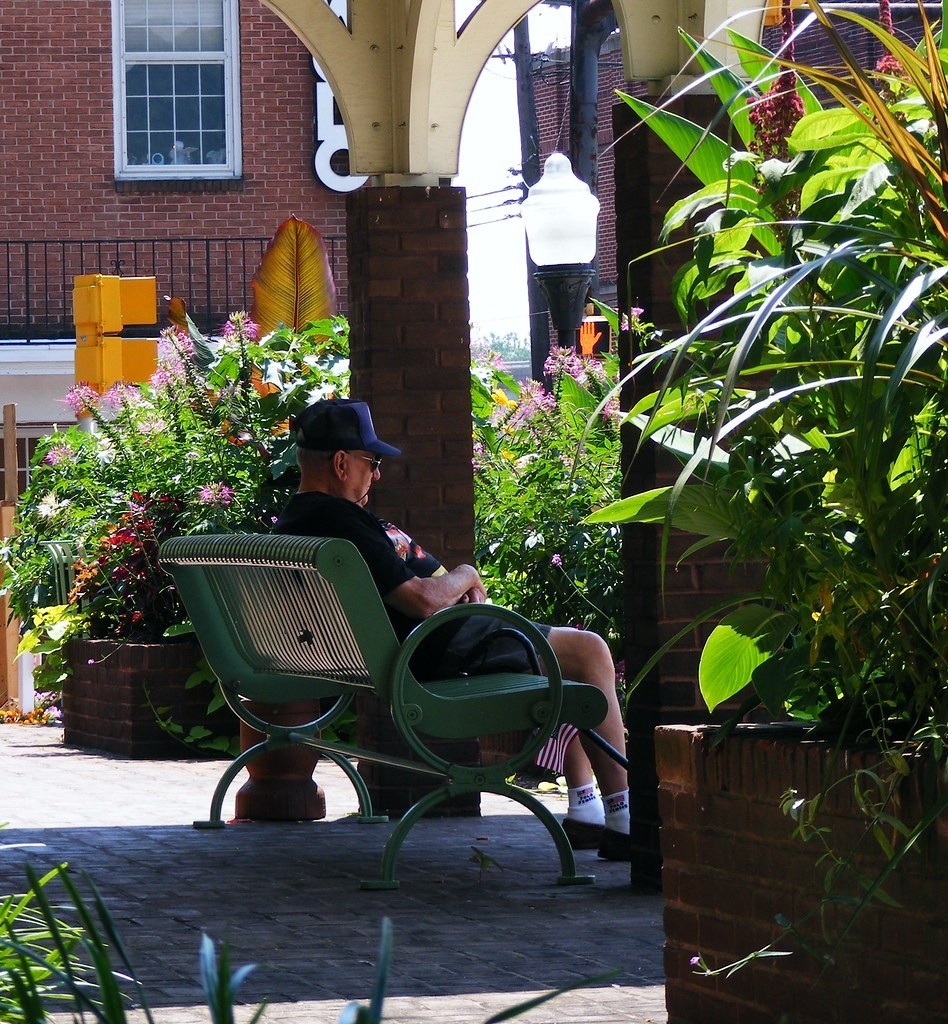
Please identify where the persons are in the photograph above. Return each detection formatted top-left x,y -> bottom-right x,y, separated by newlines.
270,399 -> 630,859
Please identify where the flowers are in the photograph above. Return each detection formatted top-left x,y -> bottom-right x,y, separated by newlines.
635,0 -> 948,741
10,288 -> 293,640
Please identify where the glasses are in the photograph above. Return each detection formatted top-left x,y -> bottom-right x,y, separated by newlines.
351,453 -> 383,472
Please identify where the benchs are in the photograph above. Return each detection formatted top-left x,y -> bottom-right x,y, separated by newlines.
159,532 -> 609,891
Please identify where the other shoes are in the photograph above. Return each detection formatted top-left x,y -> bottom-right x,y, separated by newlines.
598,828 -> 631,861
562,818 -> 602,851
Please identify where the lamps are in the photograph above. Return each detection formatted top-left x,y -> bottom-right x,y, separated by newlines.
519,150 -> 600,368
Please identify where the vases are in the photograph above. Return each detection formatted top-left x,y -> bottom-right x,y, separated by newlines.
65,632 -> 242,763
655,726 -> 948,1024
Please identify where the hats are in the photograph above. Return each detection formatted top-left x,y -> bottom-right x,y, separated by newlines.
294,399 -> 401,456
